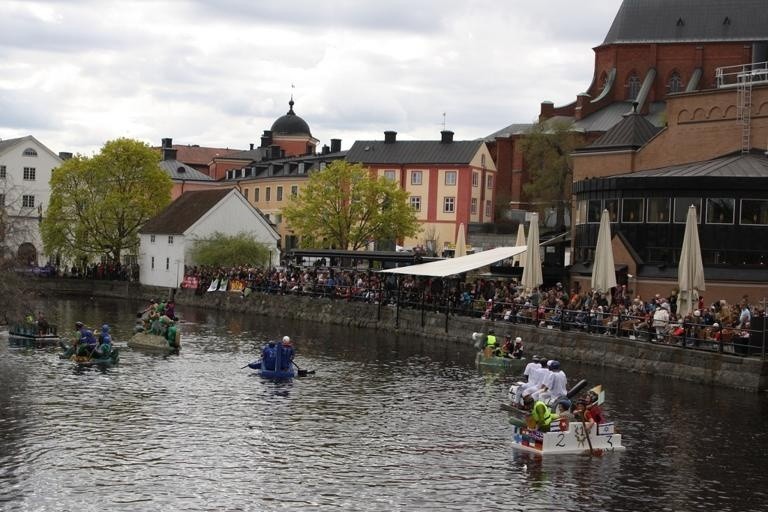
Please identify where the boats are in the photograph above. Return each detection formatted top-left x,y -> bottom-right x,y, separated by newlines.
58,339 -> 120,367
476,349 -> 526,370
248,341 -> 296,377
500,380 -> 571,417
510,418 -> 625,455
126,317 -> 176,358
10,320 -> 60,341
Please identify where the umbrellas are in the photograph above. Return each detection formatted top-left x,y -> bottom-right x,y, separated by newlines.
453,222 -> 466,258
511,223 -> 526,268
520,213 -> 542,292
675,205 -> 705,320
589,209 -> 617,306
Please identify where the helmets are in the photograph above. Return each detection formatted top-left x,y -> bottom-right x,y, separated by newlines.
268,335 -> 290,349
487,328 -> 523,344
532,355 -> 560,368
76,321 -> 111,343
523,397 -> 534,406
557,400 -> 571,411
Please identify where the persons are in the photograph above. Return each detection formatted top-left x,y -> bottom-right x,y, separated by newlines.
58,320 -> 114,363
276,336 -> 295,371
182,264 -> 448,319
261,340 -> 276,370
42,260 -> 140,283
12,311 -> 50,338
508,355 -> 606,436
132,298 -> 179,347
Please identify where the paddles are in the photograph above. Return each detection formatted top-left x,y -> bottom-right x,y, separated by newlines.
136,311 -> 147,318
